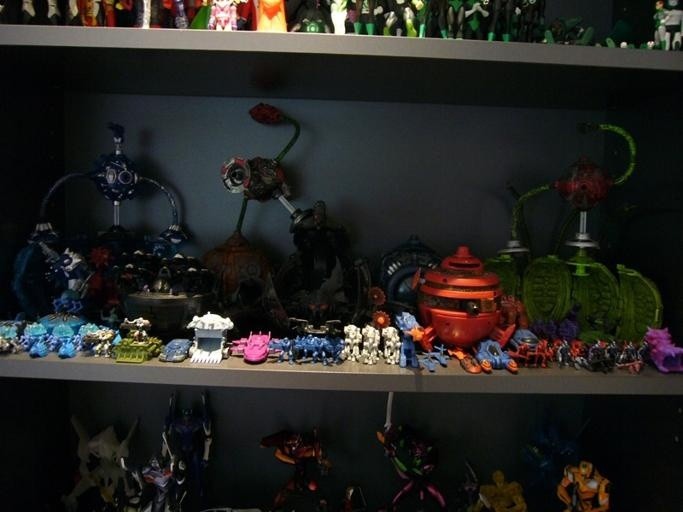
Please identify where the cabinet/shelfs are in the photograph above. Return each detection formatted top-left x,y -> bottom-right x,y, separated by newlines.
0,23 -> 681,512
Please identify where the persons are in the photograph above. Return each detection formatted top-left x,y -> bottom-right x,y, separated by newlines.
0,0 -> 668,54
